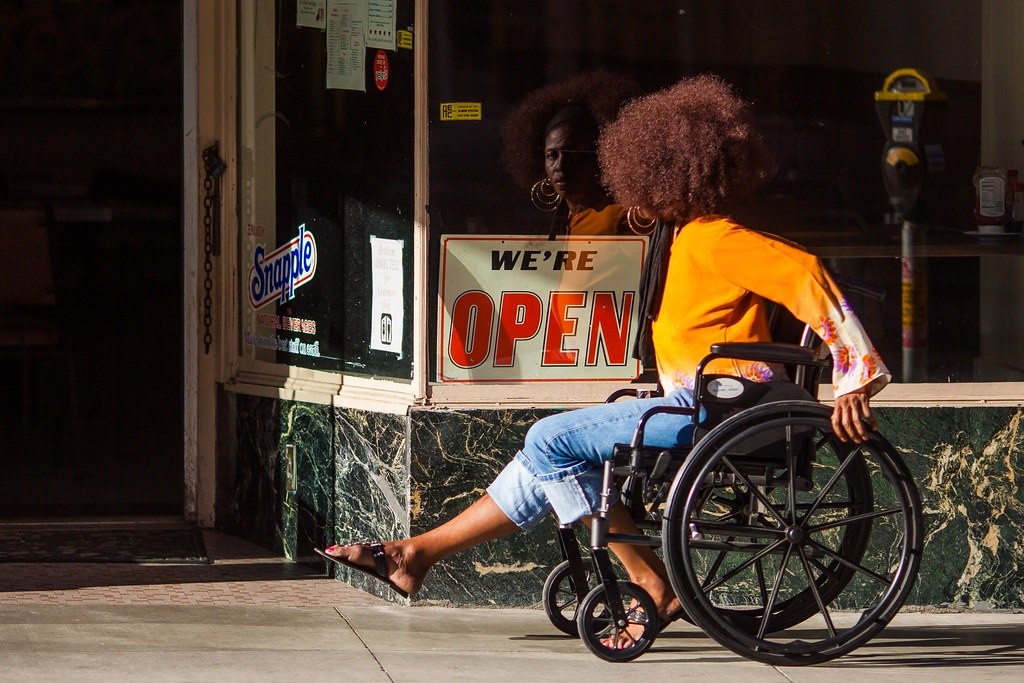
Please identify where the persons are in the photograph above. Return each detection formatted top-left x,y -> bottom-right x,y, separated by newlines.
316,75 -> 892,656
507,74 -> 653,362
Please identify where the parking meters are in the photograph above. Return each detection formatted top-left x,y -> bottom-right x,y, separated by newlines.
871,67 -> 948,385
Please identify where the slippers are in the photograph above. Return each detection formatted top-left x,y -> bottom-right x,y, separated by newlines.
314,542 -> 409,599
602,607 -> 685,649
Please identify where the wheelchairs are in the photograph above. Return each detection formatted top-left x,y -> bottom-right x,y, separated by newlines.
543,282 -> 925,667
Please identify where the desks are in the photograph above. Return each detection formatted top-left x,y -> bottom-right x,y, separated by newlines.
772,219 -> 1024,261
50,203 -> 178,436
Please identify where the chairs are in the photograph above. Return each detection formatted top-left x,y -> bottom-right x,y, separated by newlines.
0,205 -> 80,450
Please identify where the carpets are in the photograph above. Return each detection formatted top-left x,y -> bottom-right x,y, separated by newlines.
1,532 -> 209,564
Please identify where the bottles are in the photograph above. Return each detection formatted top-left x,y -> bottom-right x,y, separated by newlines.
970,166 -> 1010,235
1004,168 -> 1024,234
